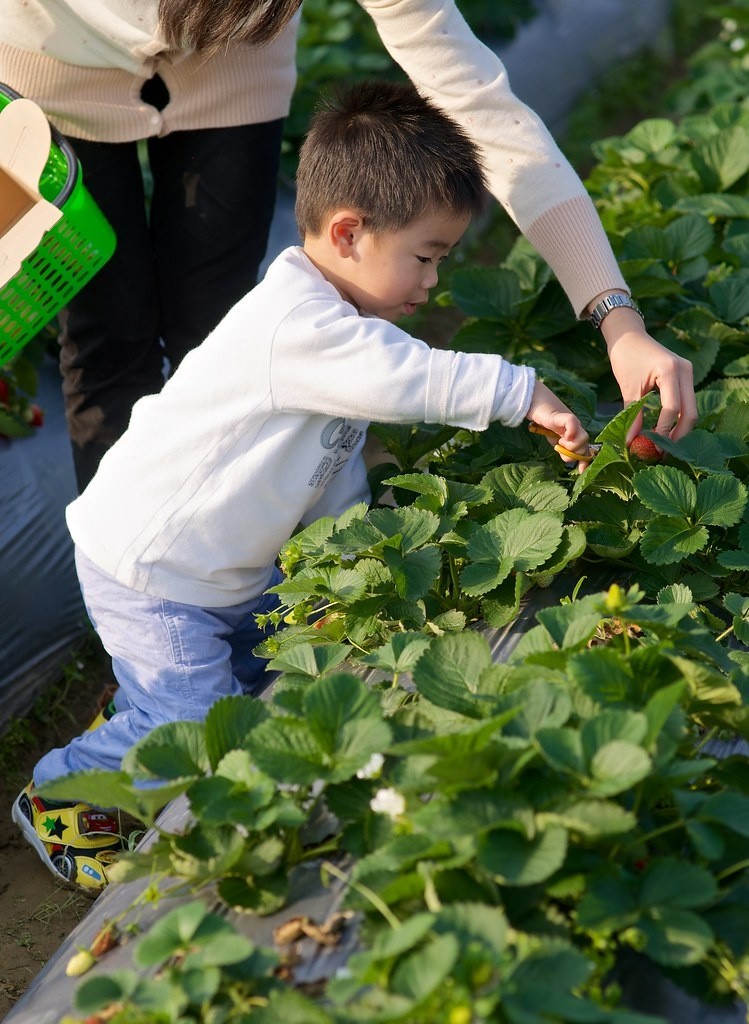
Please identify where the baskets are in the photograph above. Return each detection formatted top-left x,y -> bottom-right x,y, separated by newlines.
0,81 -> 118,370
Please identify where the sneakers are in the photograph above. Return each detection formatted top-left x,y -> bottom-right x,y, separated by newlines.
87,701 -> 115,733
11,779 -> 127,893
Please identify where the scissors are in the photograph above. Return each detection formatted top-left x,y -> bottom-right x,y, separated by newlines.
526,417 -> 603,462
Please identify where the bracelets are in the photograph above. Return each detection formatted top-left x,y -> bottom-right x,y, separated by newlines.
589,293 -> 646,329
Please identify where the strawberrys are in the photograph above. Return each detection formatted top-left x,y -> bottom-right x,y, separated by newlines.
629,428 -> 662,463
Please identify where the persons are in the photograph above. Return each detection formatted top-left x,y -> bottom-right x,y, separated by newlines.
10,82 -> 594,896
0,1 -> 696,495
